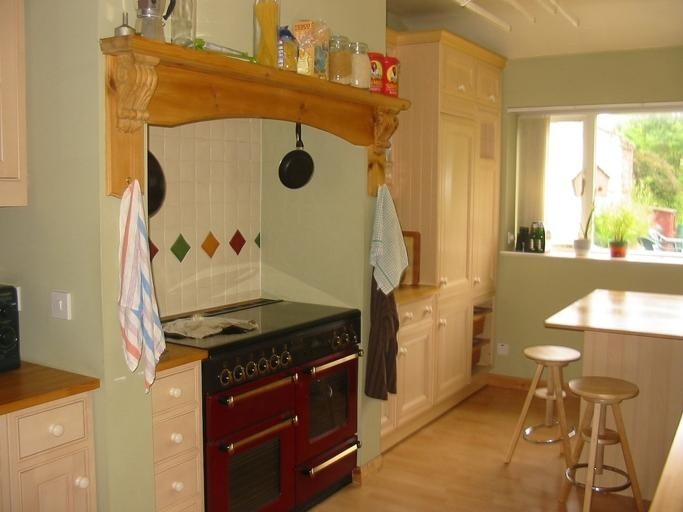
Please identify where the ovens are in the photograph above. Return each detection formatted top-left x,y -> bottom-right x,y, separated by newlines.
202,342 -> 363,512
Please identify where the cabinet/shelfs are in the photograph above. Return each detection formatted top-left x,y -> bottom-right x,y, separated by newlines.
372,284 -> 436,452
154,342 -> 209,510
436,280 -> 497,416
157,299 -> 360,509
391,25 -> 507,282
0,377 -> 100,510
0,0 -> 28,205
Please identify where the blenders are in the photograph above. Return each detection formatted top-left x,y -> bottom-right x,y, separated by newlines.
135,1 -> 178,46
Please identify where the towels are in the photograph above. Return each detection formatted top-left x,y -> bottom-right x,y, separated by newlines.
370,181 -> 408,292
113,179 -> 168,386
158,312 -> 257,337
363,277 -> 400,400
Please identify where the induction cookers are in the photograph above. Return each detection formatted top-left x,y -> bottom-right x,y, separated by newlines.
156,298 -> 362,393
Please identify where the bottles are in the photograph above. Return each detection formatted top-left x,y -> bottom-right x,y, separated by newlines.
252,0 -> 399,97
114,12 -> 136,36
516,222 -> 545,253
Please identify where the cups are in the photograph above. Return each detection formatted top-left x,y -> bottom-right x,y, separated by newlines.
170,0 -> 197,49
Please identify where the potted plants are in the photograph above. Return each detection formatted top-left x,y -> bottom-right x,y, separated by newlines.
591,201 -> 650,258
572,207 -> 596,256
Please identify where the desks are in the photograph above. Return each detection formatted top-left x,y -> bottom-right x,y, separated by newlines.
542,287 -> 682,500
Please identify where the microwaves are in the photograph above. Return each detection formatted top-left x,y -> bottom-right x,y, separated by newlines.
0,282 -> 21,371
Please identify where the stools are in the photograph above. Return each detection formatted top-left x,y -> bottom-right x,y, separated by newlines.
560,375 -> 644,511
503,346 -> 580,466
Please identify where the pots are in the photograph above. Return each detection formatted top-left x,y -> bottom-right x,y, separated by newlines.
278,120 -> 314,190
147,150 -> 166,221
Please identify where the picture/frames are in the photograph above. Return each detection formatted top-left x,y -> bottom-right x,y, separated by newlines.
395,230 -> 421,290
